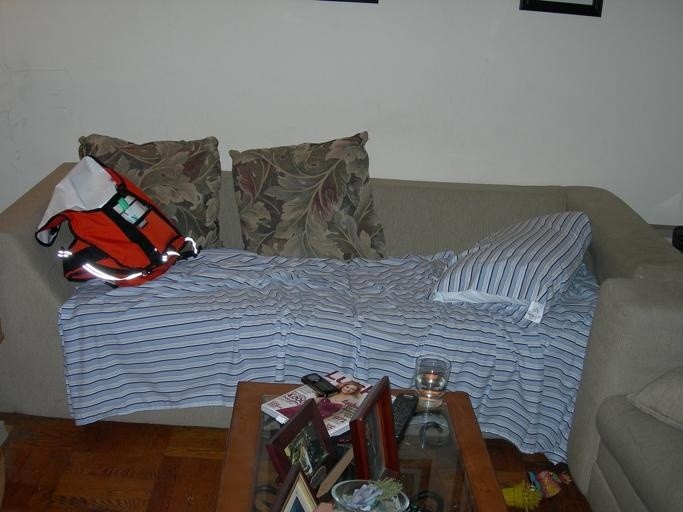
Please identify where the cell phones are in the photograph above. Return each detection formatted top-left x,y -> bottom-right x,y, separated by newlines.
301,373 -> 340,397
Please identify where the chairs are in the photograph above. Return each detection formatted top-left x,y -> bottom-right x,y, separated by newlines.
564,277 -> 683,510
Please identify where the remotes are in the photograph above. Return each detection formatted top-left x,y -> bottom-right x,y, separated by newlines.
392,393 -> 419,440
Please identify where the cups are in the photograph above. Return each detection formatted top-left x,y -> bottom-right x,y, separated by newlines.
414,353 -> 452,398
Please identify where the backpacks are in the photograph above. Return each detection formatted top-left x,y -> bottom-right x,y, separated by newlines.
33,154 -> 203,288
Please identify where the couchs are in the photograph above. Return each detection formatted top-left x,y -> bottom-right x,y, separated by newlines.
0,162 -> 683,497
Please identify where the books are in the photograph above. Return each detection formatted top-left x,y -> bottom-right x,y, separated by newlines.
261,370 -> 397,438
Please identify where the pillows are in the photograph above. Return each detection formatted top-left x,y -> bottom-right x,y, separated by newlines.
628,365 -> 683,428
228,131 -> 388,262
429,211 -> 592,310
79,133 -> 225,252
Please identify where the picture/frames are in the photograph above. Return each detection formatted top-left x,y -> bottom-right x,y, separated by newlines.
313,376 -> 403,498
517,1 -> 604,18
266,461 -> 319,512
264,396 -> 341,494
